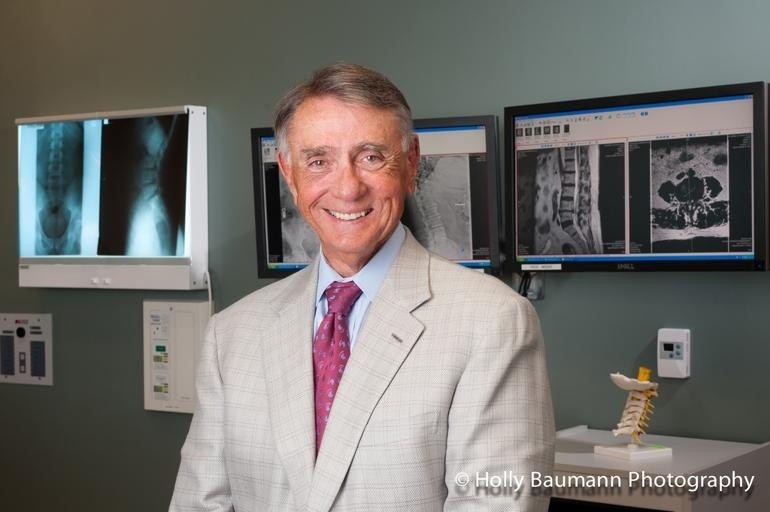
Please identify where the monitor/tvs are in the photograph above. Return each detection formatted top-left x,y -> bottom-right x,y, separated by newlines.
504,80 -> 770,272
249,114 -> 504,279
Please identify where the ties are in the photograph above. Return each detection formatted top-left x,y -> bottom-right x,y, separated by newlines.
311,280 -> 365,461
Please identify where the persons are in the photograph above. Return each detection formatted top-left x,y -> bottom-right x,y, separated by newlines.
164,63 -> 556,512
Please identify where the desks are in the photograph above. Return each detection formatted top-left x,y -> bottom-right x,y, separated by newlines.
550,423 -> 769,512
